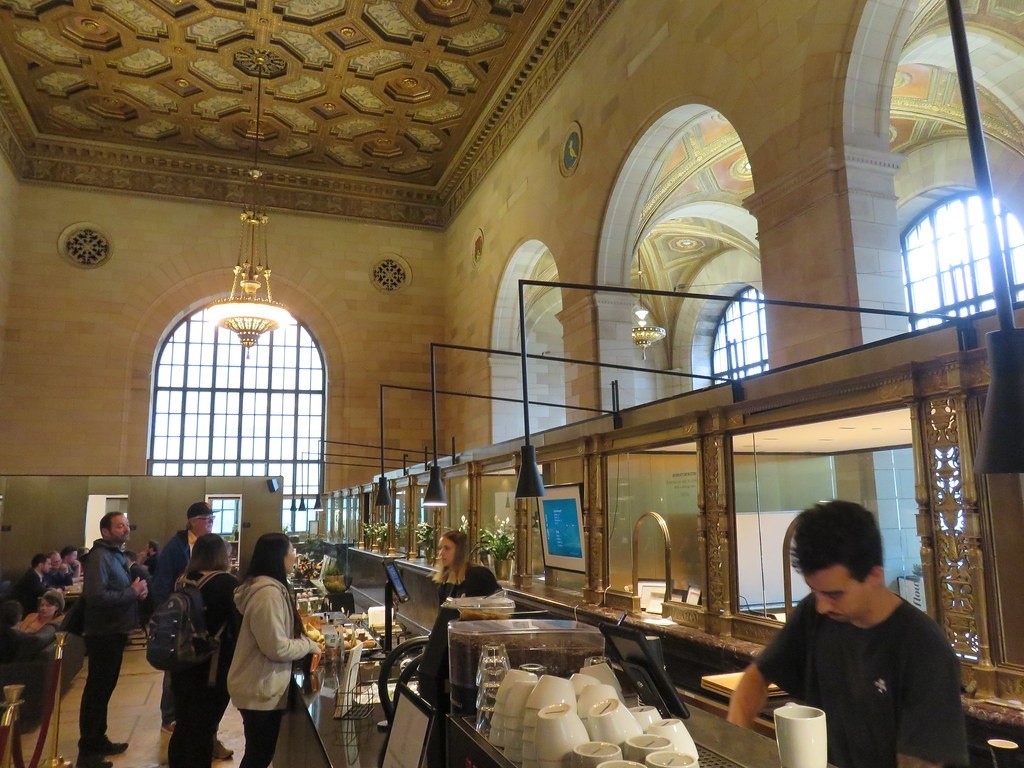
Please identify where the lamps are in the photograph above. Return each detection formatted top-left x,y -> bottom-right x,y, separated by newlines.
313,437 -> 459,511
632,249 -> 666,360
290,453 -> 409,511
515,280 -> 980,499
206,50 -> 291,360
298,445 -> 428,511
945,0 -> 1024,475
374,382 -> 624,507
422,341 -> 747,507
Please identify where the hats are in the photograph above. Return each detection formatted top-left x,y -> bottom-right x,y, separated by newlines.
43,590 -> 65,612
187,502 -> 219,520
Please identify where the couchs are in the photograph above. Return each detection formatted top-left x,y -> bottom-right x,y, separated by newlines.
0,584 -> 86,725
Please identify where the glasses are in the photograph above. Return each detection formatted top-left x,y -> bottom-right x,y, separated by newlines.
193,516 -> 216,521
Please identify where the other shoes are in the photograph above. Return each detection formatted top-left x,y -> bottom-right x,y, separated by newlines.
102,738 -> 128,754
77,756 -> 113,768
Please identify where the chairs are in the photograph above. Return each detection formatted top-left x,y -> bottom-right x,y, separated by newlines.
128,595 -> 153,646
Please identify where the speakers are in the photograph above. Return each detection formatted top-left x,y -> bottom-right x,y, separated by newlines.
266,479 -> 280,493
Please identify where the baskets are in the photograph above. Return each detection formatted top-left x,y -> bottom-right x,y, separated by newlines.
333,713 -> 375,765
332,663 -> 375,719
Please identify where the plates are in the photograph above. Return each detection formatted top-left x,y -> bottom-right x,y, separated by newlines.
343,629 -> 383,655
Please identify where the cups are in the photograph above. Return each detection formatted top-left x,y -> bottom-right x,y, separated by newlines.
772,702 -> 828,768
476,642 -> 700,768
987,738 -> 1019,768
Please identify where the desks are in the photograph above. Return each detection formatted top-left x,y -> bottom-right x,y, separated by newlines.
37,594 -> 80,613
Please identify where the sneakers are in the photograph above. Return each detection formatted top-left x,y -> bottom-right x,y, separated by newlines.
212,737 -> 233,759
162,721 -> 176,735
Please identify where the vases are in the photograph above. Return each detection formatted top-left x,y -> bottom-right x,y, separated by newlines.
494,560 -> 512,581
377,539 -> 387,554
365,538 -> 373,552
424,547 -> 434,566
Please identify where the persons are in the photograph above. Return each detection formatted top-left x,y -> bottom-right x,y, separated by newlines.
167,533 -> 248,768
0,540 -> 161,768
436,530 -> 503,611
75,512 -> 148,768
152,501 -> 215,735
727,499 -> 970,768
227,532 -> 322,768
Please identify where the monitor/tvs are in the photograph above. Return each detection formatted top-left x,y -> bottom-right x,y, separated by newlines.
381,558 -> 411,604
597,623 -> 690,719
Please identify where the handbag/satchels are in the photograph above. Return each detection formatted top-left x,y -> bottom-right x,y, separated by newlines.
57,595 -> 84,636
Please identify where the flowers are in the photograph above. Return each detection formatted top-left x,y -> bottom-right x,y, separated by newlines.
470,516 -> 516,561
443,515 -> 470,534
414,522 -> 434,550
373,523 -> 400,542
360,522 -> 380,540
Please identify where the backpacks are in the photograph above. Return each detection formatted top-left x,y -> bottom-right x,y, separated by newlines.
146,570 -> 236,672
